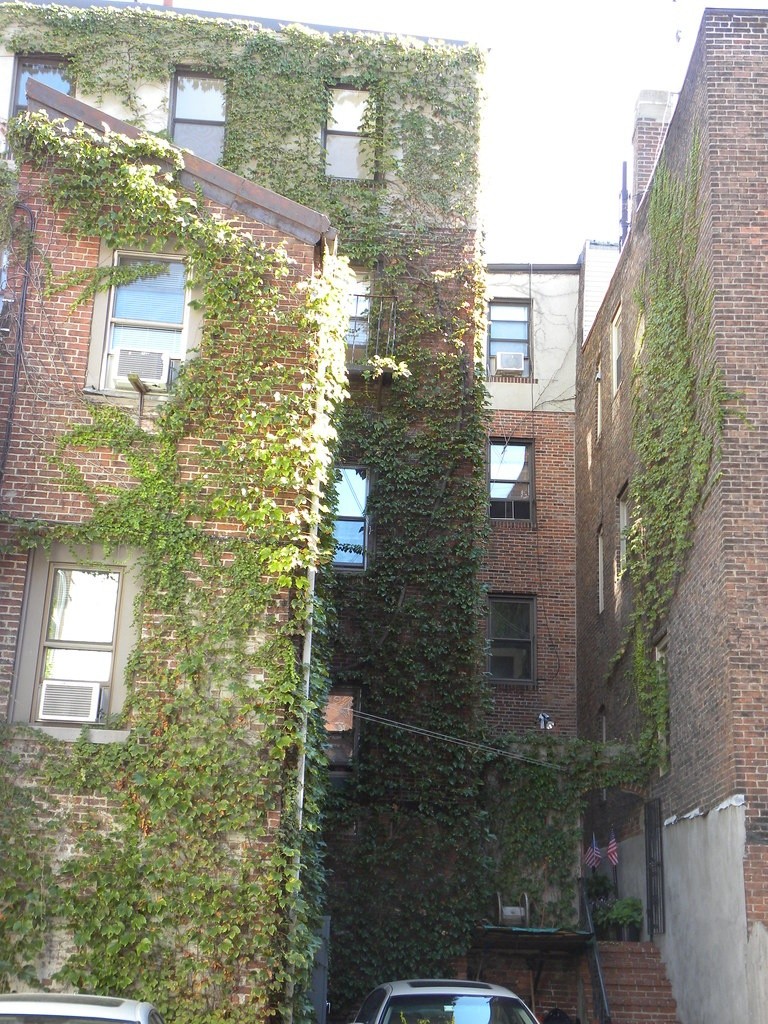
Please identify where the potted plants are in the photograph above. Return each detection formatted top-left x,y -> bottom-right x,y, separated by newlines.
607,894 -> 641,944
594,909 -> 619,942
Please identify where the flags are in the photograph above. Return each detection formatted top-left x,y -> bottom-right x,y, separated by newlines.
584,832 -> 602,870
607,826 -> 619,865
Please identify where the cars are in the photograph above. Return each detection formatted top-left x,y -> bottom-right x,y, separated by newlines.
352,978 -> 540,1024
1,991 -> 165,1024
